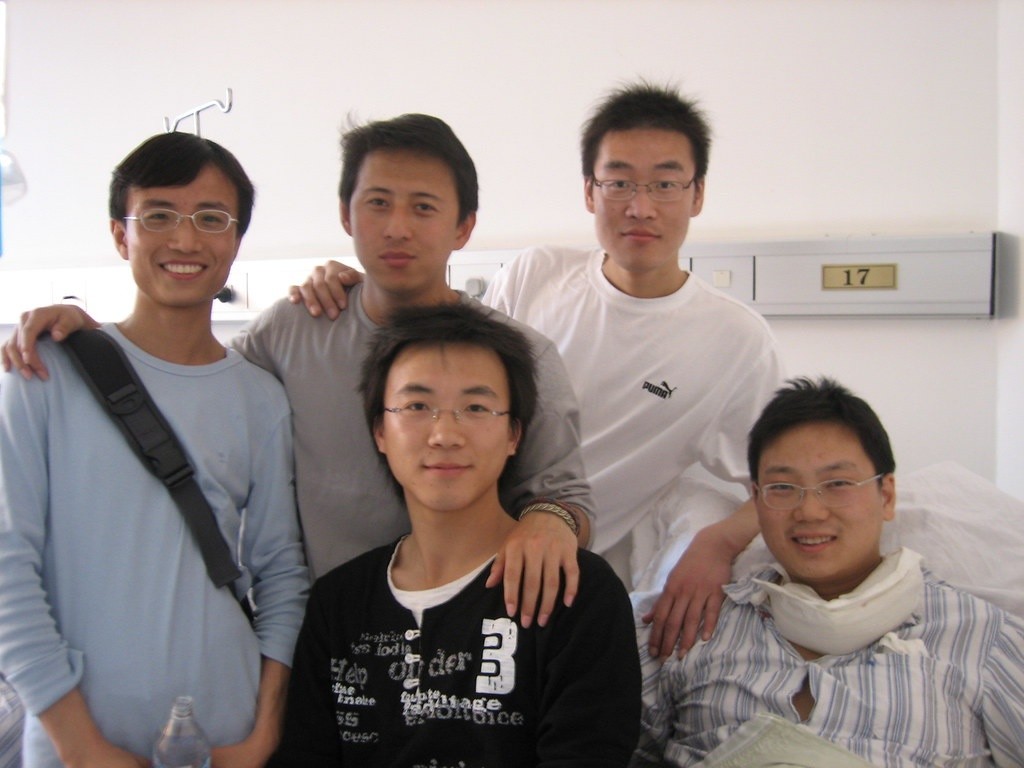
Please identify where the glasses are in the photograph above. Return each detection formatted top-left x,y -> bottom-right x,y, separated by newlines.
123,208 -> 240,233
754,473 -> 884,510
594,176 -> 695,201
384,400 -> 510,427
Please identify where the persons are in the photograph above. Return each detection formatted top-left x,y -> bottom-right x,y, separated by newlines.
288,82 -> 783,661
0,112 -> 597,628
0,131 -> 307,768
635,376 -> 1024,767
269,303 -> 642,768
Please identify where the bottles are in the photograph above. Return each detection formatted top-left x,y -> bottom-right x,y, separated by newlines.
151,696 -> 212,768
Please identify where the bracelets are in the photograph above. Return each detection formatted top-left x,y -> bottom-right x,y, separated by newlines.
519,497 -> 581,536
